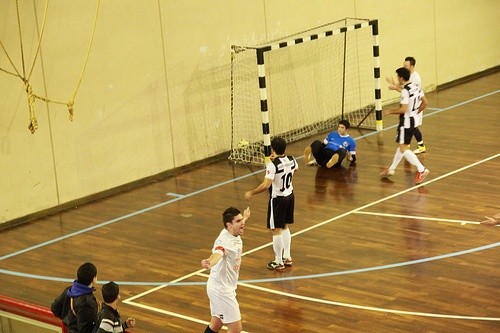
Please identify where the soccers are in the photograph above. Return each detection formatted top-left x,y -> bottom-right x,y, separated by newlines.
236,138 -> 249,150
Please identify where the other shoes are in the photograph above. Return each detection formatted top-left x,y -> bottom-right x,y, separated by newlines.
304,146 -> 311,162
326,153 -> 339,168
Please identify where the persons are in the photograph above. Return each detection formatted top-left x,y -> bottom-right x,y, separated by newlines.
379,67 -> 430,184
91,281 -> 136,333
386,57 -> 427,153
245,136 -> 299,272
51,262 -> 98,333
201,207 -> 251,333
306,120 -> 356,169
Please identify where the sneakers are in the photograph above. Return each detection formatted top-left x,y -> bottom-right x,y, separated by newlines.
267,261 -> 286,270
282,257 -> 293,265
413,145 -> 426,154
416,168 -> 430,183
379,170 -> 394,177
